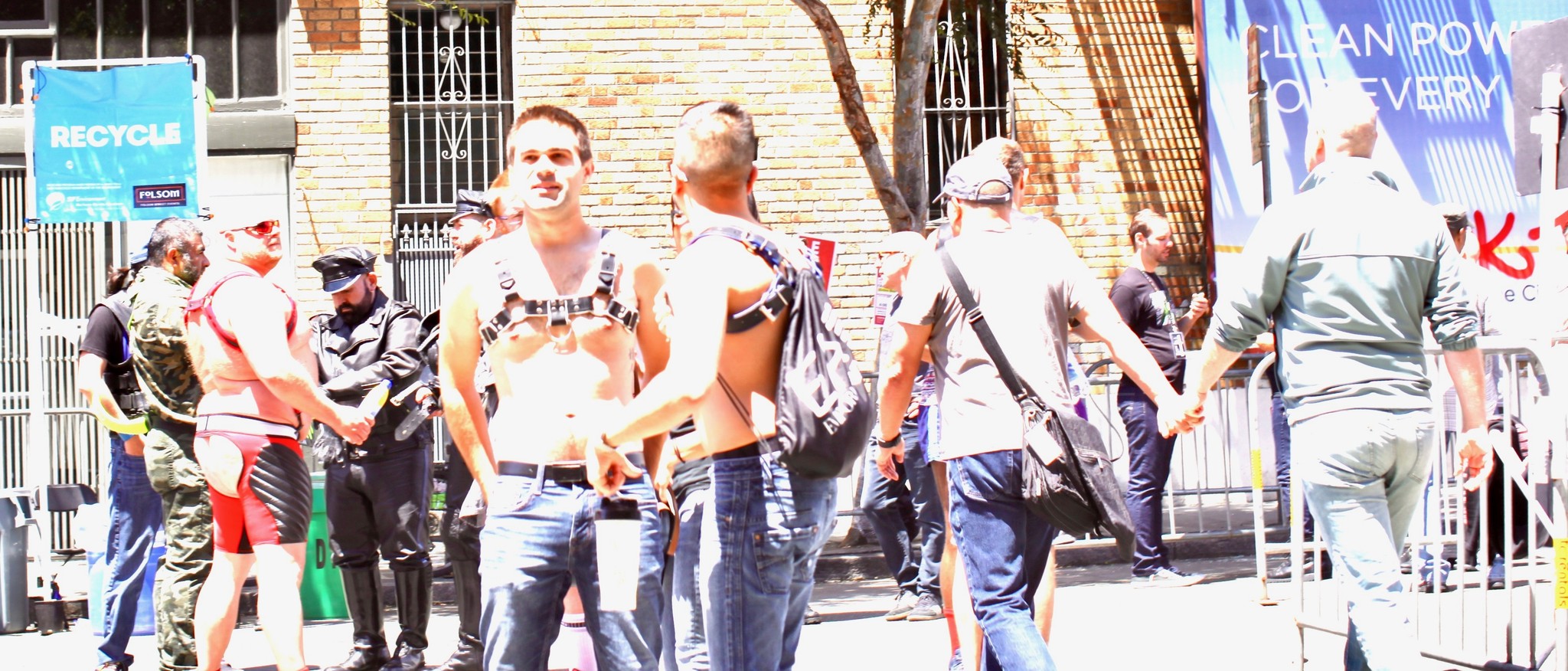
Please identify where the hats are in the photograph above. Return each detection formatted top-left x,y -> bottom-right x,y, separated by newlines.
448,188 -> 494,225
312,246 -> 377,294
1434,202 -> 1475,232
932,153 -> 1015,205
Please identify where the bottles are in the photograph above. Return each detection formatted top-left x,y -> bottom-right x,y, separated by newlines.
357,380 -> 391,420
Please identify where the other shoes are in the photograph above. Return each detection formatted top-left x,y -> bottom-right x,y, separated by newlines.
1454,564 -> 1479,571
218,660 -> 244,671
1409,580 -> 1448,593
1487,580 -> 1505,590
1401,559 -> 1412,573
95,660 -> 129,671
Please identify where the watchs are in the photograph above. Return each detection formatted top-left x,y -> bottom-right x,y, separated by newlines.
876,433 -> 902,448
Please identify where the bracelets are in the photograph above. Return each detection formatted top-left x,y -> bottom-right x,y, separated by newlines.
118,433 -> 135,443
600,432 -> 618,450
672,437 -> 686,464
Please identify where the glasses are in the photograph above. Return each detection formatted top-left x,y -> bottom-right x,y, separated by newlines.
877,251 -> 904,259
219,219 -> 280,235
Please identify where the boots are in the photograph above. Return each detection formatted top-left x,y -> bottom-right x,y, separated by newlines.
378,563 -> 432,671
431,559 -> 484,671
324,566 -> 390,671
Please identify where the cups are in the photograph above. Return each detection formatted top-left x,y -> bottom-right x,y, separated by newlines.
593,496 -> 642,611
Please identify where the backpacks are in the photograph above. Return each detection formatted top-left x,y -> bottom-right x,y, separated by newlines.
716,225 -> 878,479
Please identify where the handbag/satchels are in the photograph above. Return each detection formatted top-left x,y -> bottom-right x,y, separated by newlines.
1020,396 -> 1136,545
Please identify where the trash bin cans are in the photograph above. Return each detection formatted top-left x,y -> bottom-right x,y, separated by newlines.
0,488 -> 43,635
299,471 -> 350,624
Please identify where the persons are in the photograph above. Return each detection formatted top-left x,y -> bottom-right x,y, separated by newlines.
1182,90 -> 1494,670
127,218 -> 214,671
308,247 -> 435,670
875,154 -> 1183,671
860,136 -> 1207,671
78,240 -> 164,671
183,202 -> 374,671
414,100 -> 837,671
1253,203 -> 1532,592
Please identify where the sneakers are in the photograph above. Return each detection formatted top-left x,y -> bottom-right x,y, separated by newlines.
1266,538 -> 1332,583
948,648 -> 964,671
1130,567 -> 1209,588
884,589 -> 946,621
802,605 -> 822,625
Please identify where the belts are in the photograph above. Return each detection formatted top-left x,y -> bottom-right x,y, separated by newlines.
196,415 -> 299,440
497,451 -> 647,484
710,434 -> 783,462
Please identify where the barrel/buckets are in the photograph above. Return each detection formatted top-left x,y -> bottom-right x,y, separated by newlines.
299,472 -> 353,624
0,496 -> 89,633
72,504 -> 167,636
